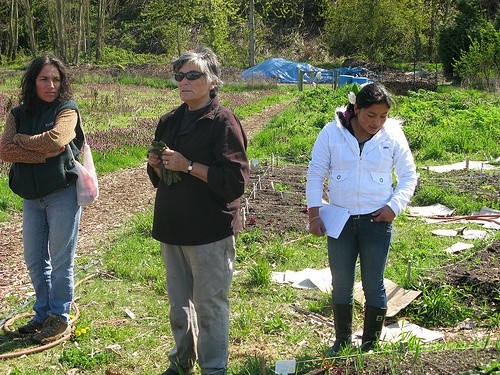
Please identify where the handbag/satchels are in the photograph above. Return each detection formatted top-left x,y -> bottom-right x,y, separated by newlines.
68,113 -> 99,207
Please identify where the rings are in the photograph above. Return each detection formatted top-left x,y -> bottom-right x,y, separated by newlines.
165,165 -> 168,169
165,161 -> 169,165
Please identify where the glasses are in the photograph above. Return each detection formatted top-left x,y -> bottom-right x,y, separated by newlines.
175,70 -> 206,81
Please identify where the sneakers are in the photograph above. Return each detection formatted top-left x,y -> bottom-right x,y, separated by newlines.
19,320 -> 44,332
33,317 -> 73,344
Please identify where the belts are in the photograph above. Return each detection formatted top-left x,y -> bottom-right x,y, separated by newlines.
351,215 -> 373,220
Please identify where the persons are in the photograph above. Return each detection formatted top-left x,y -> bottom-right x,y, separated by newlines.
305,83 -> 419,360
147,48 -> 250,375
0,55 -> 84,345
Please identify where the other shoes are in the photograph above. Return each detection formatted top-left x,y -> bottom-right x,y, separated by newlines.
161,368 -> 180,375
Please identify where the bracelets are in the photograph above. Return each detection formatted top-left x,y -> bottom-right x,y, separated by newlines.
308,216 -> 320,224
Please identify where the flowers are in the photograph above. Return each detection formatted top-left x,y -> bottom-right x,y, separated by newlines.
344,83 -> 358,106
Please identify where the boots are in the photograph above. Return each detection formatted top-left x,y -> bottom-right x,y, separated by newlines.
360,305 -> 387,352
329,302 -> 354,354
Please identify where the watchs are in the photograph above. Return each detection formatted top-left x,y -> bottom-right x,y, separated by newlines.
187,161 -> 194,176
